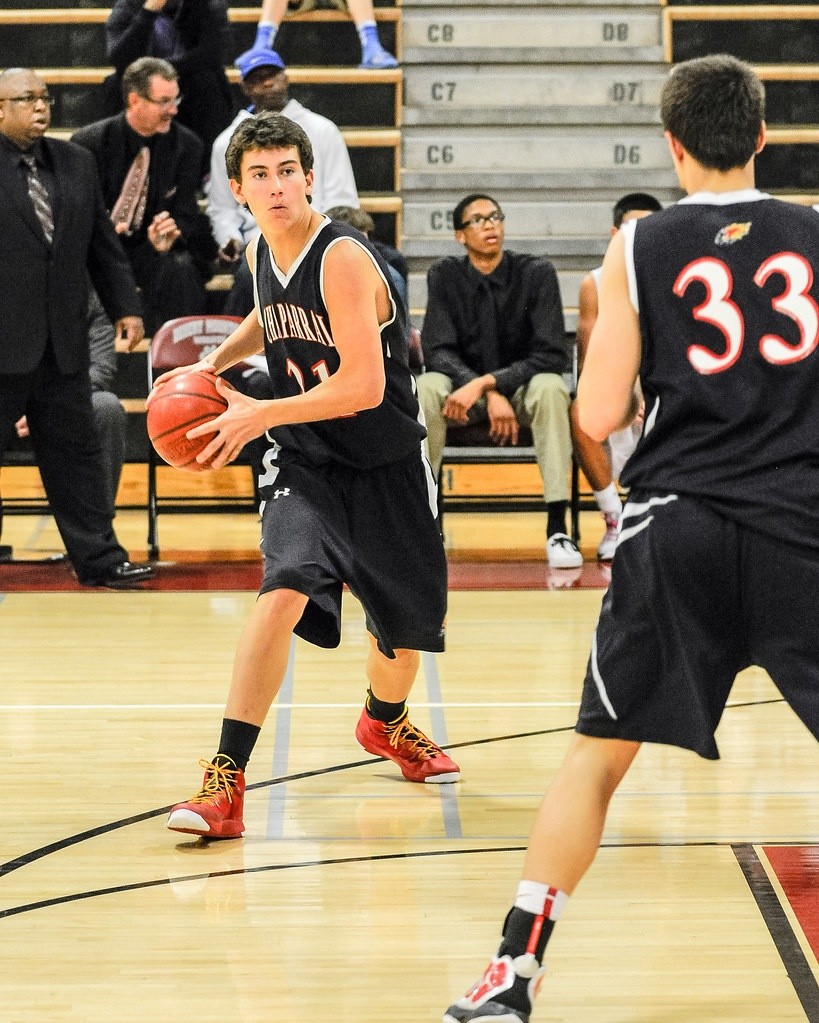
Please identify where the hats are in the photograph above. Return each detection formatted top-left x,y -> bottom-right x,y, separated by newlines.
239,49 -> 285,82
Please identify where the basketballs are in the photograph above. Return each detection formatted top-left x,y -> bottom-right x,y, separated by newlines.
143,370 -> 237,472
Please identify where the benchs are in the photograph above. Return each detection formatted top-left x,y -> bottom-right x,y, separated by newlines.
0,0 -> 819,421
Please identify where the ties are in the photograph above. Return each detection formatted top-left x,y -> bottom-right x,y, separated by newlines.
21,153 -> 55,247
107,147 -> 150,235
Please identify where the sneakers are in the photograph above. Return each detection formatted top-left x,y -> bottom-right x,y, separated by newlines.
167,753 -> 245,838
234,44 -> 272,68
445,949 -> 545,1023
596,509 -> 621,561
356,705 -> 461,784
546,532 -> 583,568
358,50 -> 399,69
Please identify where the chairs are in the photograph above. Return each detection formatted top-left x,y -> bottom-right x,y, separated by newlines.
1,315 -> 634,573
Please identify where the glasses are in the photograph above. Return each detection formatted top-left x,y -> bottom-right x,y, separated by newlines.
457,209 -> 506,230
1,91 -> 57,108
145,92 -> 183,110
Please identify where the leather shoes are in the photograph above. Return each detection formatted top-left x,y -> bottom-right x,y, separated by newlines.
99,561 -> 156,587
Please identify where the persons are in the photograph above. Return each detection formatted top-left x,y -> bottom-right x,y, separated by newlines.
12,271 -> 128,578
168,110 -> 460,838
245,208 -> 413,506
68,55 -> 216,334
569,194 -> 667,563
233,0 -> 400,69
450,52 -> 819,1023
203,49 -> 358,314
417,193 -> 584,567
101,0 -> 231,143
0,67 -> 156,589
